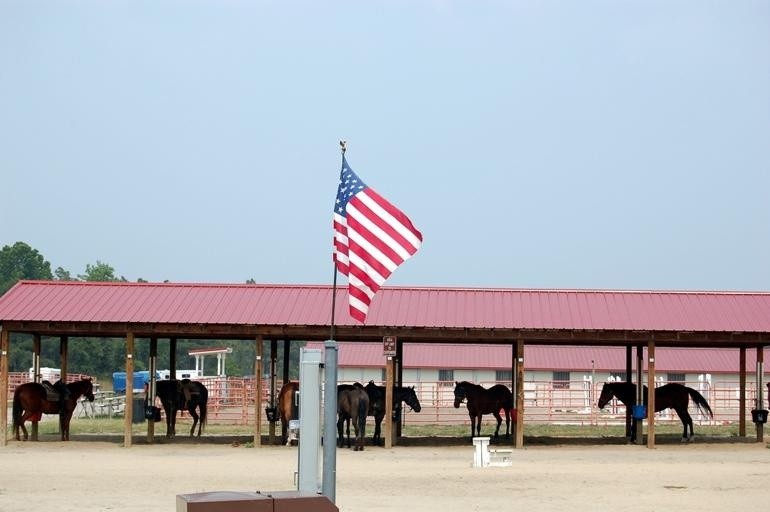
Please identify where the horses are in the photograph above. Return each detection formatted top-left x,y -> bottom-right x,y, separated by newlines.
13,376 -> 95,441
278,382 -> 299,447
453,380 -> 512,438
351,386 -> 421,442
337,385 -> 369,450
143,380 -> 208,439
597,381 -> 712,444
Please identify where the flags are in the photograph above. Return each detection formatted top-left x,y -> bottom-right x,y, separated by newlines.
332,151 -> 424,327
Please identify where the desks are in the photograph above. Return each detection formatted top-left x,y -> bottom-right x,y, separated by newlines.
93,391 -> 115,396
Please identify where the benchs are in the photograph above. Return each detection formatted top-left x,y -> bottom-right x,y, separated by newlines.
79,397 -> 125,418
